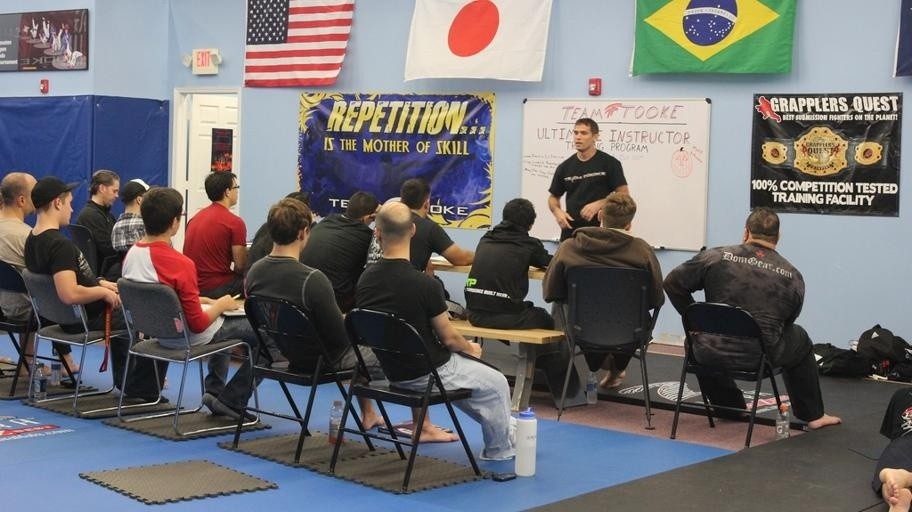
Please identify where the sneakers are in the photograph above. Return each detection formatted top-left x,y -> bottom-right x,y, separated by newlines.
203,391 -> 220,415
211,398 -> 261,423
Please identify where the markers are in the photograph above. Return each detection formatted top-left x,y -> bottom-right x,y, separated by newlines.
650,246 -> 665,249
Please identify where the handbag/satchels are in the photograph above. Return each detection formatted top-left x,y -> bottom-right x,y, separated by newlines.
813,324 -> 912,383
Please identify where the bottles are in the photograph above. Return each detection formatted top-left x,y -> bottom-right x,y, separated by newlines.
33,347 -> 61,402
328,400 -> 346,454
587,371 -> 598,404
775,403 -> 791,440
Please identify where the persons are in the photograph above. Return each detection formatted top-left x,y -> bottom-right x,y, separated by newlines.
355,202 -> 515,461
244,197 -> 385,430
463,198 -> 556,330
1,168 -> 171,403
662,206 -> 842,430
547,118 -> 632,242
122,187 -> 278,424
543,192 -> 664,389
183,169 -> 474,321
872,386 -> 912,512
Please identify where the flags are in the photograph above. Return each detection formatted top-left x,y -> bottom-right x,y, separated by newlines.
629,0 -> 798,75
244,0 -> 357,88
403,1 -> 553,82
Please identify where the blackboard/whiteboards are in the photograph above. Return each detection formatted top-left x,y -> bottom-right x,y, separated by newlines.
520,98 -> 712,253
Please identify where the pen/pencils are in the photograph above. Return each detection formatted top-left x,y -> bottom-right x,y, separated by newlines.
232,293 -> 242,299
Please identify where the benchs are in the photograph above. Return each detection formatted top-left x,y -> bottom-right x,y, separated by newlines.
440,315 -> 564,418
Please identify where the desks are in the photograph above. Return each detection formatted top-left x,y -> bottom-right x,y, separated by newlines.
427,260 -> 547,286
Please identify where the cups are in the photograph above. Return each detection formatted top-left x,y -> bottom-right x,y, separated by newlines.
40,79 -> 49,95
514,406 -> 538,476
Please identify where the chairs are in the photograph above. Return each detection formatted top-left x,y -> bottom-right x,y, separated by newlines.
235,293 -> 375,464
330,307 -> 482,490
116,275 -> 261,434
22,268 -> 162,415
668,302 -> 787,449
556,265 -> 655,431
0,260 -> 82,396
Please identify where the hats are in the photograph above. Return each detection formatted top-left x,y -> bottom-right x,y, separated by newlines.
32,177 -> 81,209
121,178 -> 159,200
